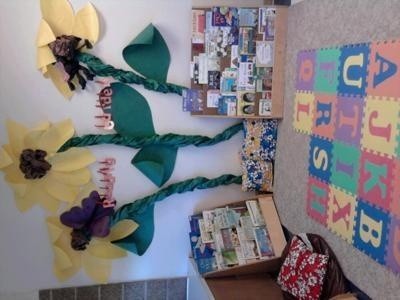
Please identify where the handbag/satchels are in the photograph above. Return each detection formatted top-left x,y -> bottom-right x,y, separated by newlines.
276,233 -> 330,300
